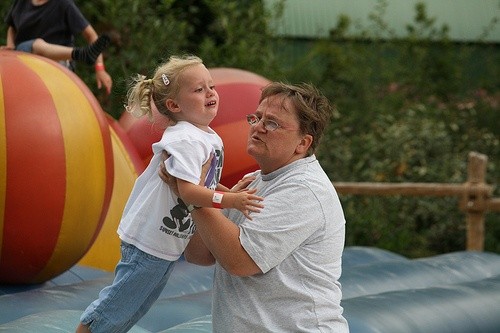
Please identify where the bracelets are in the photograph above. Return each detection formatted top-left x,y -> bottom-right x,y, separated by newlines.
94,64 -> 105,70
212,191 -> 224,208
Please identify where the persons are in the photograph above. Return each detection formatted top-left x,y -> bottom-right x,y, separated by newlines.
6,0 -> 113,95
158,79 -> 351,333
74,53 -> 263,332
16,32 -> 111,65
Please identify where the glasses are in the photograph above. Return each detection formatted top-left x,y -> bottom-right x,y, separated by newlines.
245,113 -> 303,132
